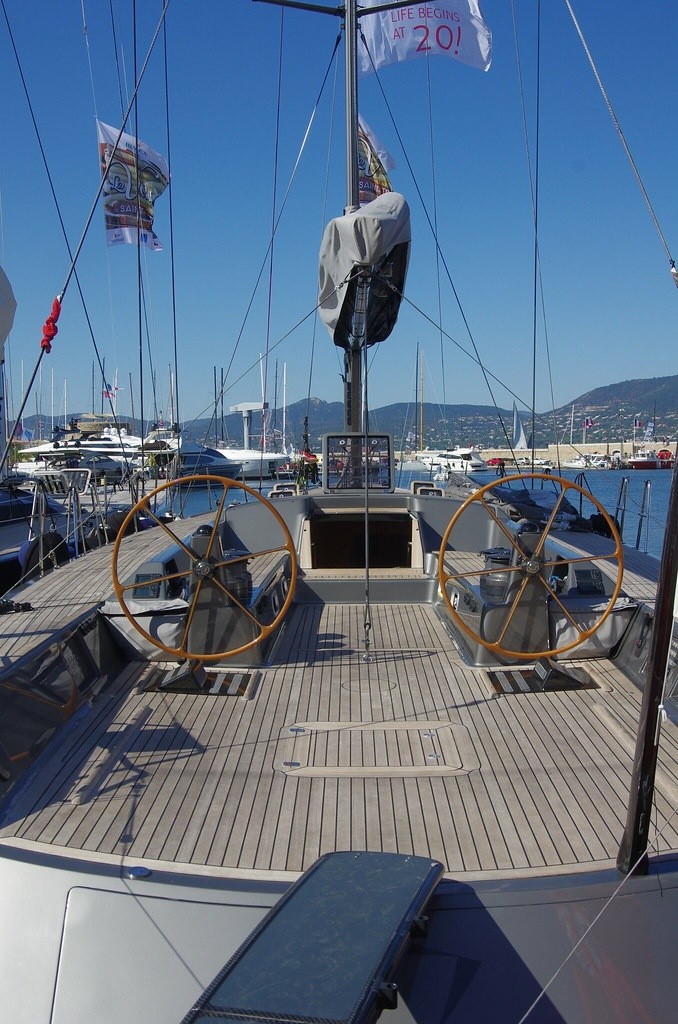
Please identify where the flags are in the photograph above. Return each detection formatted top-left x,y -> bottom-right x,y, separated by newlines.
303,451 -> 319,465
14,420 -> 33,441
583,418 -> 594,429
103,384 -> 125,398
357,0 -> 493,72
95,119 -> 171,250
358,111 -> 396,209
635,419 -> 642,428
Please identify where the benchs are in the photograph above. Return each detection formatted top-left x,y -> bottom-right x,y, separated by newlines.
247,548 -> 296,666
430,549 -> 555,666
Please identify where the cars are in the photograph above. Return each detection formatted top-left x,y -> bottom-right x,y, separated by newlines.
486,457 -> 504,467
512,457 -> 530,466
529,456 -> 550,466
500,457 -> 514,466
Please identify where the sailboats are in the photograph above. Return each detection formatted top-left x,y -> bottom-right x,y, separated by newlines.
0,2 -> 678,1024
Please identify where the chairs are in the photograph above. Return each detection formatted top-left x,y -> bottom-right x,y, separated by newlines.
268,483 -> 300,498
411,481 -> 446,498
